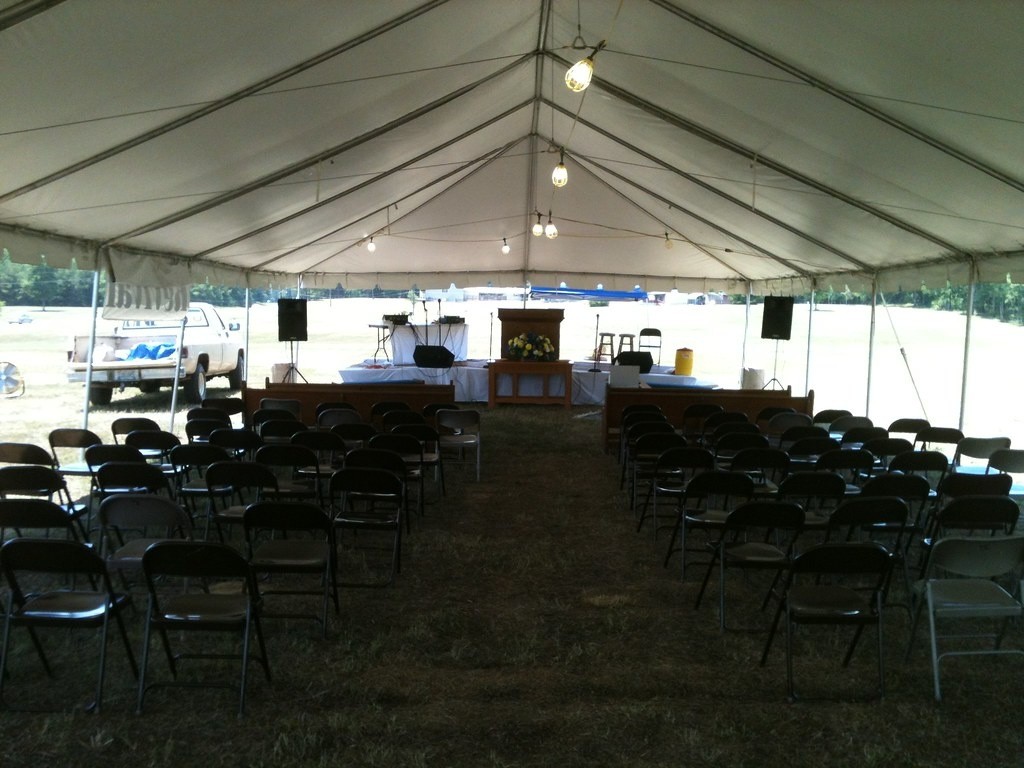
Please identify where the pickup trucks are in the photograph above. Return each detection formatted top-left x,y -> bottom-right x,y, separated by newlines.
67,301 -> 247,406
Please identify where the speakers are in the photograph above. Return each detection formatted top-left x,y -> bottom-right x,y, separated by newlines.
278,298 -> 307,341
762,296 -> 794,340
612,352 -> 653,373
413,345 -> 455,368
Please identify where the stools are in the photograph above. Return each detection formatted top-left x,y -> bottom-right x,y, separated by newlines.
617,333 -> 636,358
597,331 -> 615,362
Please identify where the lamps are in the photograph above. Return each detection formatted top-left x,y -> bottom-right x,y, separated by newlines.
564,39 -> 608,93
534,209 -> 558,240
552,149 -> 568,188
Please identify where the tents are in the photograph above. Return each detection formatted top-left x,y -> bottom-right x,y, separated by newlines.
0,1 -> 1024,469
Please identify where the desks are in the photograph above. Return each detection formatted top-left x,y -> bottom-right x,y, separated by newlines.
368,322 -> 470,366
485,358 -> 574,410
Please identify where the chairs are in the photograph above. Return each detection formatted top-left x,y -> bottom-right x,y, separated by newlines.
639,328 -> 662,367
0,399 -> 483,722
620,402 -> 1024,706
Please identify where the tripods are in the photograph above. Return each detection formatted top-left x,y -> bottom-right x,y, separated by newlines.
762,340 -> 784,390
282,341 -> 308,383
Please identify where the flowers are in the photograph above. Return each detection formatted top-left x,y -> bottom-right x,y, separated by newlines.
506,330 -> 557,360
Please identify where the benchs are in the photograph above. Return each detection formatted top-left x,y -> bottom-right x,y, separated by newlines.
241,378 -> 457,427
600,383 -> 814,453
